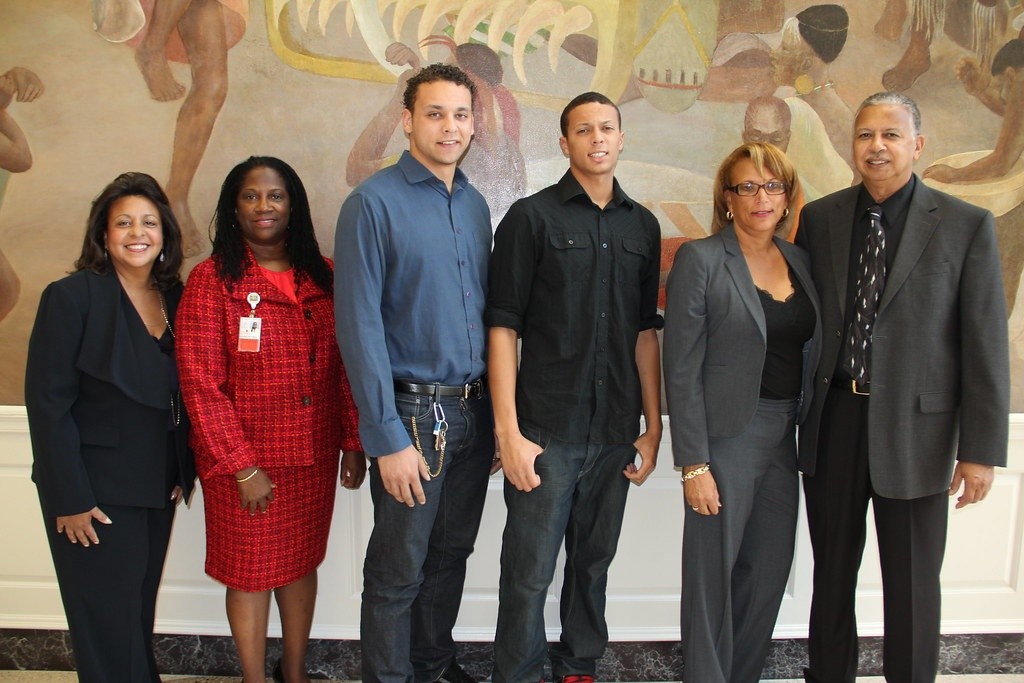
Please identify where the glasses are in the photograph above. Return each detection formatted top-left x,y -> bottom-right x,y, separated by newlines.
724,181 -> 788,196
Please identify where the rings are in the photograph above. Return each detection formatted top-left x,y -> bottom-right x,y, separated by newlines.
693,507 -> 698,511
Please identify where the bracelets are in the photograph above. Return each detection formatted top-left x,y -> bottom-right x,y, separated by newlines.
681,464 -> 710,482
236,469 -> 257,483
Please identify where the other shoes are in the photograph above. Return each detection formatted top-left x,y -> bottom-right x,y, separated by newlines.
562,674 -> 594,683
435,657 -> 474,683
272,657 -> 289,683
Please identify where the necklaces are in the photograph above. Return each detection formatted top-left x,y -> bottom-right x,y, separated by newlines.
144,272 -> 181,427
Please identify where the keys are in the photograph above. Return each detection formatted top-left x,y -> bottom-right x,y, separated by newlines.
433,420 -> 449,451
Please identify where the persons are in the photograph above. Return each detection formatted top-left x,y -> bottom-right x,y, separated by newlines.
483,92 -> 663,683
663,90 -> 1011,683
24,171 -> 198,683
333,64 -> 502,683
174,155 -> 367,683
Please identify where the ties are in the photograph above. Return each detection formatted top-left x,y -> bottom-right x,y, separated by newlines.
841,205 -> 886,385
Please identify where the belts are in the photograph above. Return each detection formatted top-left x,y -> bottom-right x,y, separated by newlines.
393,374 -> 488,401
831,376 -> 873,397
